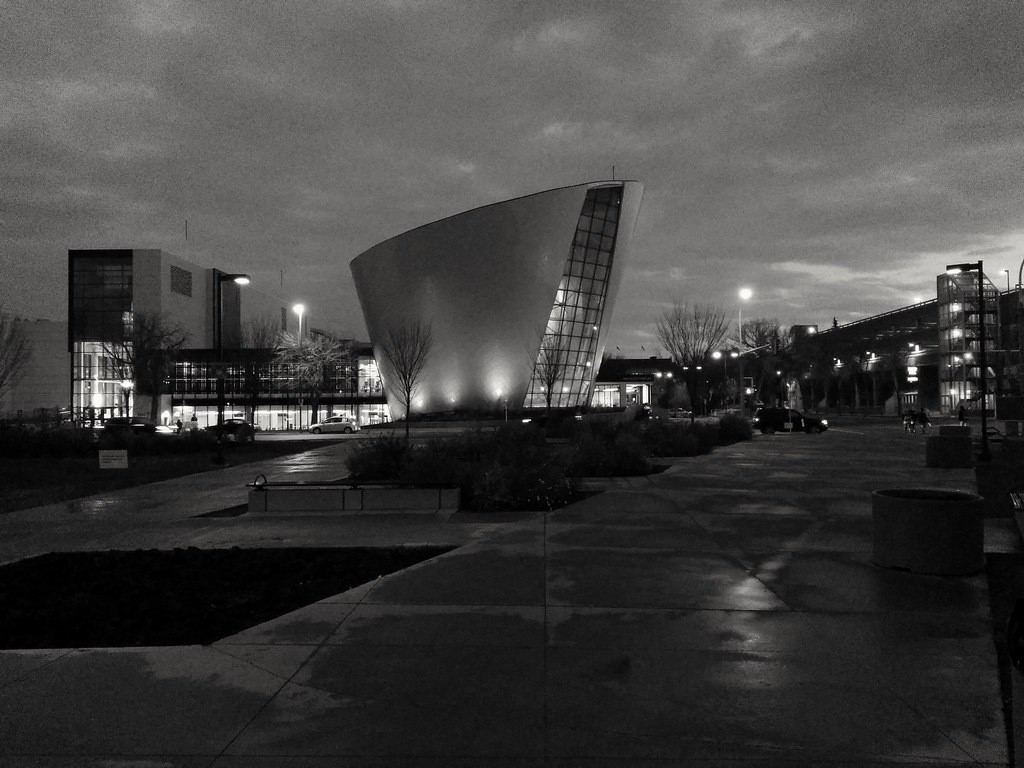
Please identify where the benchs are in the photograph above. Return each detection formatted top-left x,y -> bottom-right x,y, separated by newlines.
245,473 -> 470,513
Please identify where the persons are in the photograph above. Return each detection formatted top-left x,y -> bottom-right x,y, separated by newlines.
917,407 -> 932,435
175,419 -> 182,437
902,408 -> 917,435
689,410 -> 695,423
957,405 -> 970,427
192,414 -> 197,430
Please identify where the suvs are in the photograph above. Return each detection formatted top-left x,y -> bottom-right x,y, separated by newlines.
752,408 -> 829,435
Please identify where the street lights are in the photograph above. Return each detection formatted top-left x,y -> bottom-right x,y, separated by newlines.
744,378 -> 753,406
712,350 -> 739,415
736,285 -> 758,420
291,301 -> 309,345
216,272 -> 249,441
945,263 -> 990,480
656,372 -> 673,389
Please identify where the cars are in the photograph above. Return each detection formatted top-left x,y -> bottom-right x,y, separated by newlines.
644,406 -> 652,415
308,416 -> 362,434
106,417 -> 159,433
666,408 -> 691,418
204,418 -> 260,441
